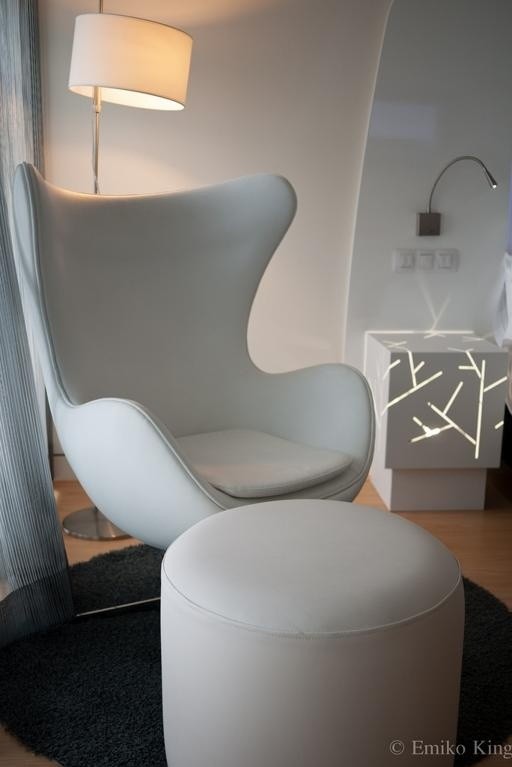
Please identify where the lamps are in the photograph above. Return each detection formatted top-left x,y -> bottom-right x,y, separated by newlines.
415,153 -> 498,236
67,12 -> 196,540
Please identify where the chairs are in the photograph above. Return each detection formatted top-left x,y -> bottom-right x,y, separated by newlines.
8,156 -> 378,619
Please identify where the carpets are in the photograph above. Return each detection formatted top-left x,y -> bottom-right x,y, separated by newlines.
2,540 -> 510,762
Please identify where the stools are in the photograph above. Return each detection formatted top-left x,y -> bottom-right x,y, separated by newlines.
158,497 -> 469,767
